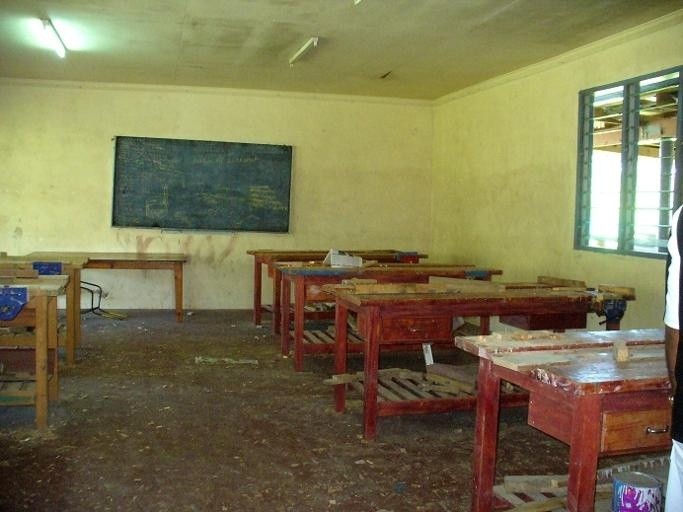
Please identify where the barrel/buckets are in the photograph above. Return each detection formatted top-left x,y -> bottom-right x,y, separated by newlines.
613,470 -> 662,512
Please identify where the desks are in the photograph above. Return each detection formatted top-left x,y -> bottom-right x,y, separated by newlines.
321,276 -> 637,441
245,248 -> 430,337
267,262 -> 504,373
454,329 -> 674,512
1,250 -> 188,431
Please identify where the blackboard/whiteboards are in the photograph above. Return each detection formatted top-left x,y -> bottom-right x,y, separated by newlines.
111,136 -> 295,235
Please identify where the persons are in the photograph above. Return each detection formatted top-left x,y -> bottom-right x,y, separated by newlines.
662,204 -> 682,511
